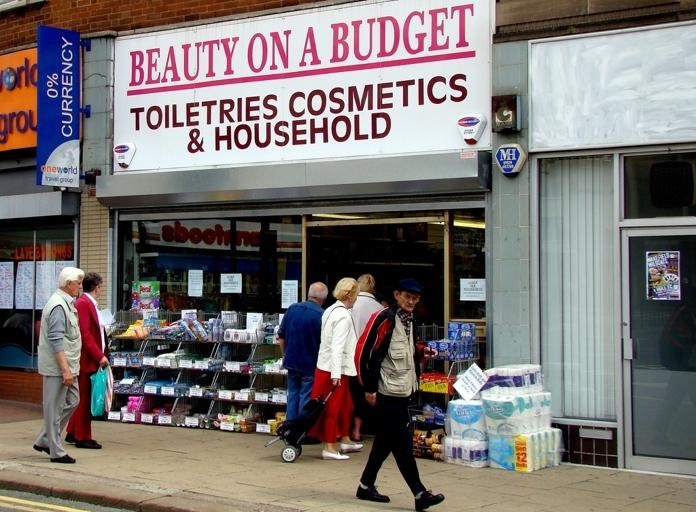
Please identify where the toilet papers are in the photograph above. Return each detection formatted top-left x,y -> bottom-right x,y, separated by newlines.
442,364 -> 562,473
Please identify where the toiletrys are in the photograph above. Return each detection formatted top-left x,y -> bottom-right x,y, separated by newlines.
181,318 -> 223,341
224,328 -> 255,342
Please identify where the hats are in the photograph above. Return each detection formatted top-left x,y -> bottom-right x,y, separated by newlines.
397,278 -> 422,293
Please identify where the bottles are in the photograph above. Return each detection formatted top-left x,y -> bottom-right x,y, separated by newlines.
179,318 -> 225,342
263,324 -> 279,344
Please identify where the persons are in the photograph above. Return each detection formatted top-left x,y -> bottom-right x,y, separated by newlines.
349,274 -> 385,441
310,277 -> 364,460
277,281 -> 329,445
354,285 -> 444,512
33,266 -> 86,463
64,272 -> 111,449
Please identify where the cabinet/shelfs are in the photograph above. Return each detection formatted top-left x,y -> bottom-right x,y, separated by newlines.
402,321 -> 483,463
99,304 -> 286,435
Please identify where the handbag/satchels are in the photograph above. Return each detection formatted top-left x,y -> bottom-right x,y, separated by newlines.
105,367 -> 114,413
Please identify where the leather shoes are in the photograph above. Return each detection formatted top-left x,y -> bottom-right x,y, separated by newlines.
76,439 -> 101,449
64,432 -> 75,442
321,449 -> 350,460
355,484 -> 391,503
415,491 -> 445,511
50,455 -> 76,463
33,444 -> 49,454
340,443 -> 364,453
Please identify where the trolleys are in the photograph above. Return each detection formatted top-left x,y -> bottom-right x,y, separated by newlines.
262,381 -> 339,463
